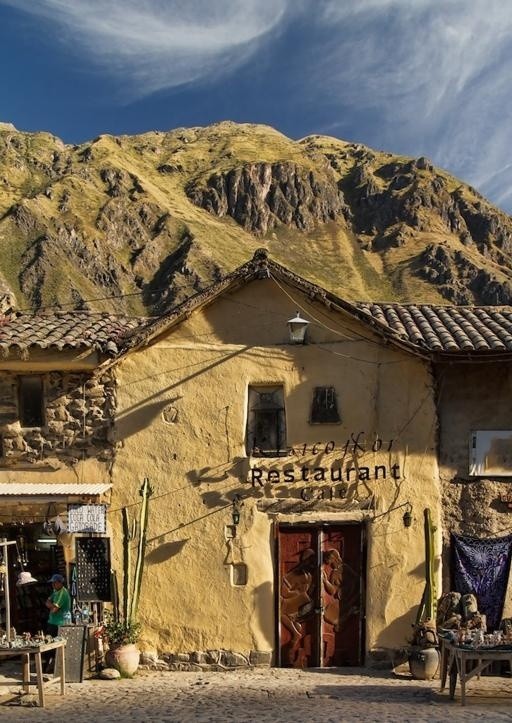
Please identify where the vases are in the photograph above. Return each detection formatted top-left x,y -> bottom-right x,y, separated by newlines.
408,645 -> 441,679
105,643 -> 140,679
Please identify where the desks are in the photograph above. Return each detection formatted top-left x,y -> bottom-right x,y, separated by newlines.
0,639 -> 67,707
440,638 -> 512,706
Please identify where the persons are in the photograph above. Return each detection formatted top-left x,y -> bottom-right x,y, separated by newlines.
41,574 -> 72,674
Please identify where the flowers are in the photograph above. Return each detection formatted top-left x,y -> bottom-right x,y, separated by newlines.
93,608 -> 141,645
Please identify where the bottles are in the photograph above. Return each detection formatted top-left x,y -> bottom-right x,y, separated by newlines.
63,605 -> 90,625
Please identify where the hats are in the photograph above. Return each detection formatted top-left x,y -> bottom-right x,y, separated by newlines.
48,574 -> 64,583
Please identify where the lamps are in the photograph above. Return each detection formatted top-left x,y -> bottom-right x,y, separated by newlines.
0,540 -> 38,588
403,501 -> 413,527
287,311 -> 312,345
232,492 -> 243,524
